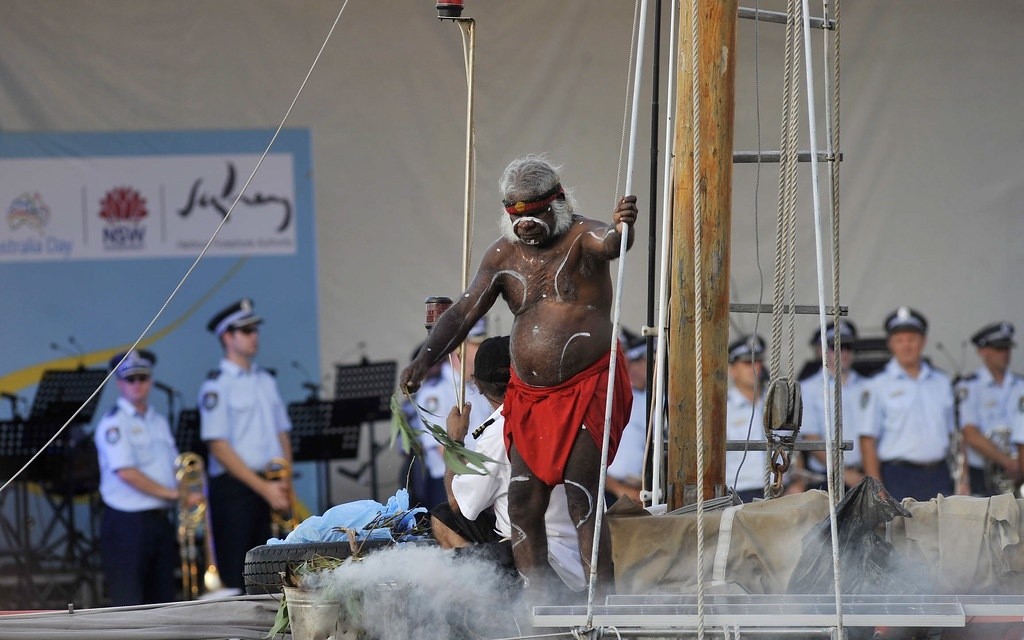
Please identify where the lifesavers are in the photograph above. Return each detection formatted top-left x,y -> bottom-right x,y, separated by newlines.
241,538 -> 439,594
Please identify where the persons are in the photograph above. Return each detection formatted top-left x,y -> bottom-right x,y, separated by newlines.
394,304 -> 1024,504
402,152 -> 638,605
199,295 -> 294,593
94,348 -> 204,607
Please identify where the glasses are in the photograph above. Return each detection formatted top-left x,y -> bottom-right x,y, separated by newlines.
126,376 -> 148,383
235,327 -> 257,334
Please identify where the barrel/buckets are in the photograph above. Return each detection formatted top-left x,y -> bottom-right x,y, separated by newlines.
284,585 -> 364,640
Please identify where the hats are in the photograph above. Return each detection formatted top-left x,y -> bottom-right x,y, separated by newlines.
882,307 -> 929,334
109,349 -> 155,379
207,299 -> 263,339
618,328 -> 658,362
727,333 -> 765,366
972,321 -> 1017,350
810,319 -> 856,350
472,336 -> 512,383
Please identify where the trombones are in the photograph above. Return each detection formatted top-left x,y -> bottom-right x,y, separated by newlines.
266,456 -> 298,540
174,453 -> 222,602
